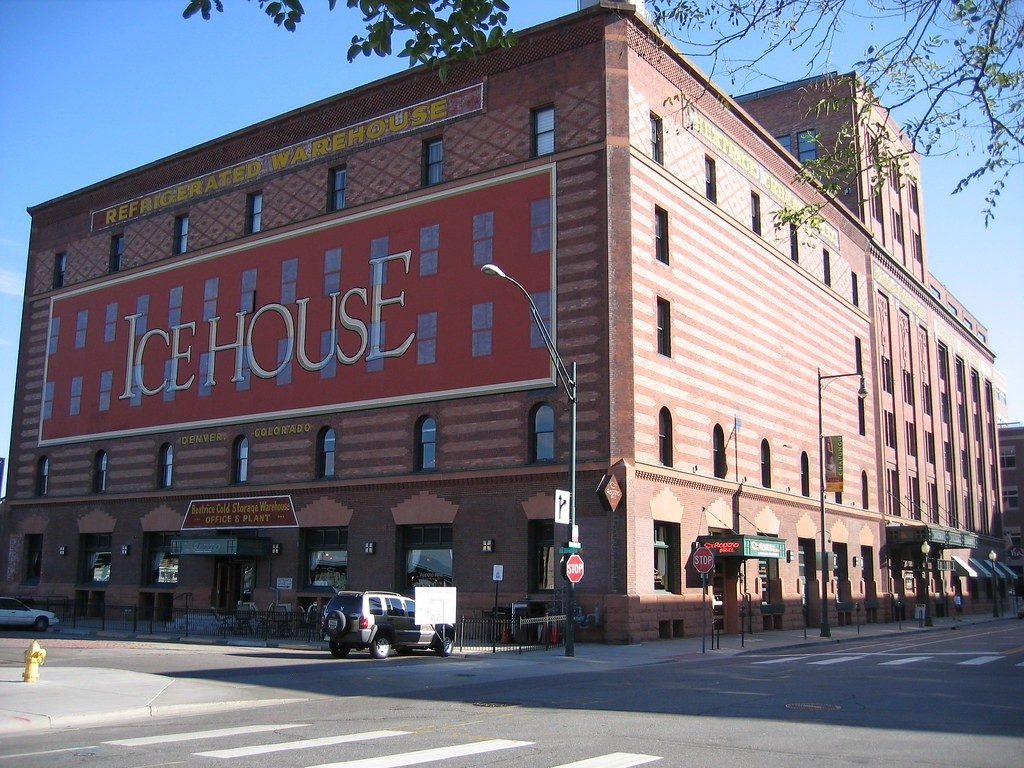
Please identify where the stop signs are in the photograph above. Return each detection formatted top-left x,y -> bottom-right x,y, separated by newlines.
693,547 -> 714,574
560,554 -> 585,583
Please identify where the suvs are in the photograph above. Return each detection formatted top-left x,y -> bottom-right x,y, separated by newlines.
321,590 -> 456,659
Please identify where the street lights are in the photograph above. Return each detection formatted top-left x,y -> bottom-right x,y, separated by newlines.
818,369 -> 867,638
921,540 -> 934,626
481,264 -> 577,659
989,550 -> 999,617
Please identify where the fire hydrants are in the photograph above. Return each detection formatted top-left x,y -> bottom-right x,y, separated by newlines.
22,640 -> 46,682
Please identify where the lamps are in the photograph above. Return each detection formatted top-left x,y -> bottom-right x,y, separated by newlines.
59,546 -> 65,556
364,542 -> 375,555
853,556 -> 859,567
482,539 -> 493,553
272,543 -> 280,554
122,545 -> 128,555
787,550 -> 795,562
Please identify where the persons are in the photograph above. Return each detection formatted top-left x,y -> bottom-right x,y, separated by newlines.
294,604 -> 306,637
306,601 -> 317,643
953,591 -> 962,622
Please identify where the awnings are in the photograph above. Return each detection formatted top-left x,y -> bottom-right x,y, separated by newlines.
968,558 -> 992,577
997,561 -> 1017,579
886,491 -> 977,549
984,560 -> 1006,578
951,556 -> 977,577
700,508 -> 785,559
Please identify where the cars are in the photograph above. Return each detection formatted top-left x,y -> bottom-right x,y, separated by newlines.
0,597 -> 59,632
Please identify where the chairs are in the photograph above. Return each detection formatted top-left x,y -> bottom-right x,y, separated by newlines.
210,601 -> 320,642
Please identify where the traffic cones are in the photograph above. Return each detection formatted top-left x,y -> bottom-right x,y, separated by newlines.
547,619 -> 561,643
498,624 -> 510,642
539,620 -> 549,643
